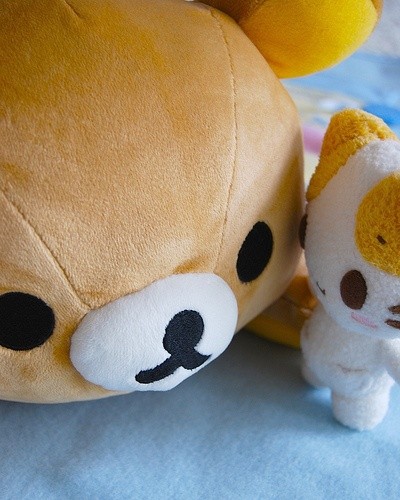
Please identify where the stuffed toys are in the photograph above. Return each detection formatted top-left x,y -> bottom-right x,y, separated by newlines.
298,109 -> 399,429
0,0 -> 382,403
